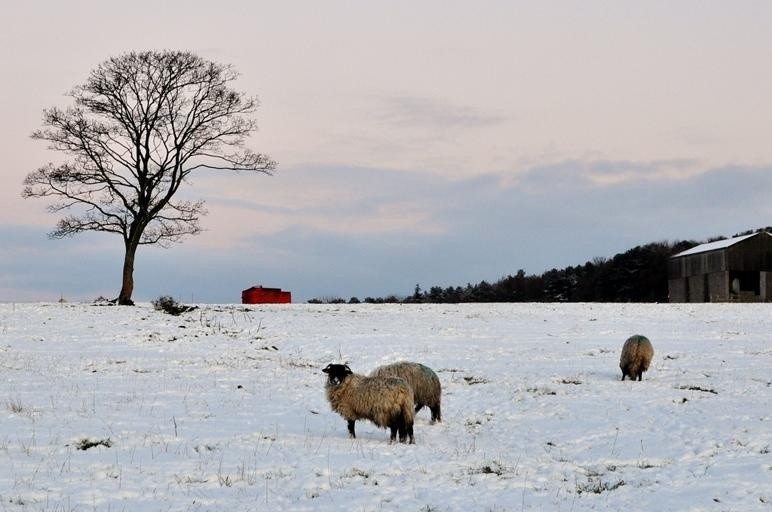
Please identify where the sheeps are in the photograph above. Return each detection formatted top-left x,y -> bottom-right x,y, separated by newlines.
619,335 -> 654,381
368,361 -> 442,425
321,362 -> 415,444
